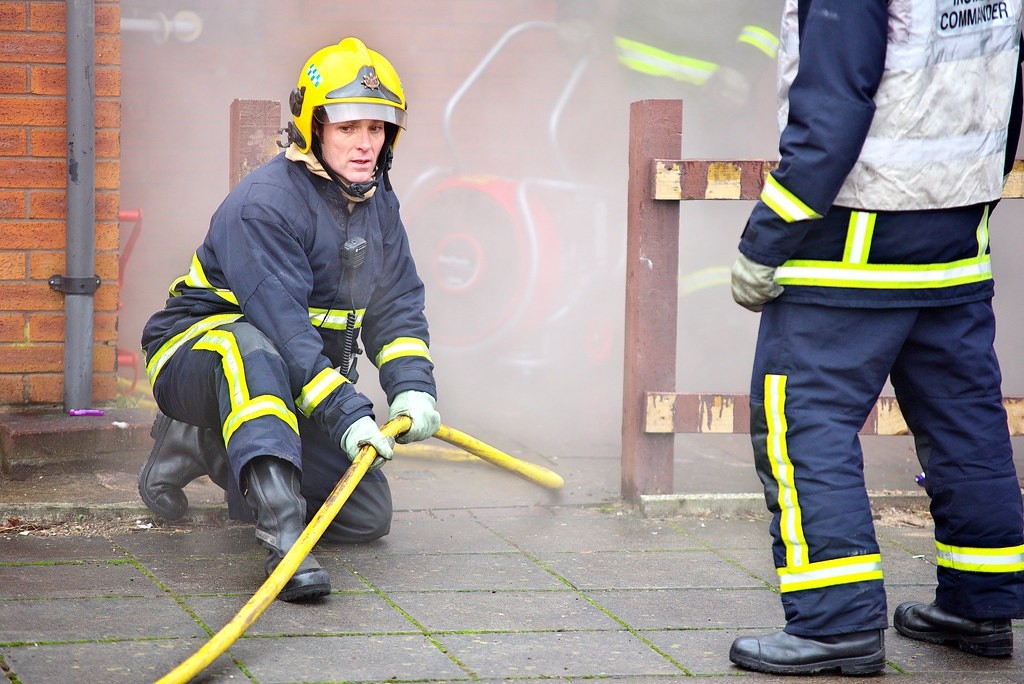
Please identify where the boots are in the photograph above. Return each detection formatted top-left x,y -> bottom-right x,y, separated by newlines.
242,455 -> 331,599
138,411 -> 228,520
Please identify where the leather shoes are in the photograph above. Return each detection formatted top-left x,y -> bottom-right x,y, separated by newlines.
893,599 -> 1015,656
729,628 -> 886,676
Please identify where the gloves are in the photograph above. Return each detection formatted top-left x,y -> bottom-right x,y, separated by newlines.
387,388 -> 440,443
340,415 -> 395,473
731,254 -> 786,312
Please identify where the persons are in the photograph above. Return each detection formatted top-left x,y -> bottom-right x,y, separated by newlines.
137,36 -> 440,598
728,0 -> 1024,679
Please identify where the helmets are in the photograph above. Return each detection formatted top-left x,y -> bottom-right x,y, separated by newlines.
286,38 -> 406,172
